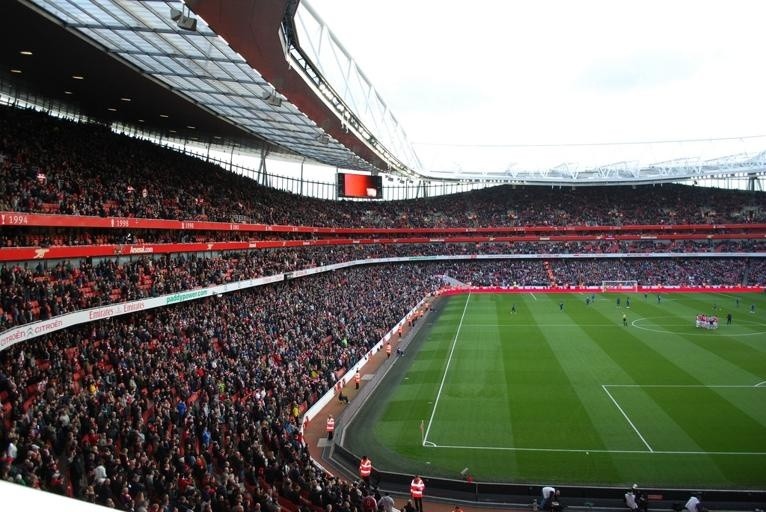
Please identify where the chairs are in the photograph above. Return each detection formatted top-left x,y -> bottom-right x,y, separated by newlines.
0,191 -> 343,511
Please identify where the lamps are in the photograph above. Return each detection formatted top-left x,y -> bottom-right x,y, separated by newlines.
168,1 -> 199,33
314,132 -> 330,146
262,87 -> 282,108
